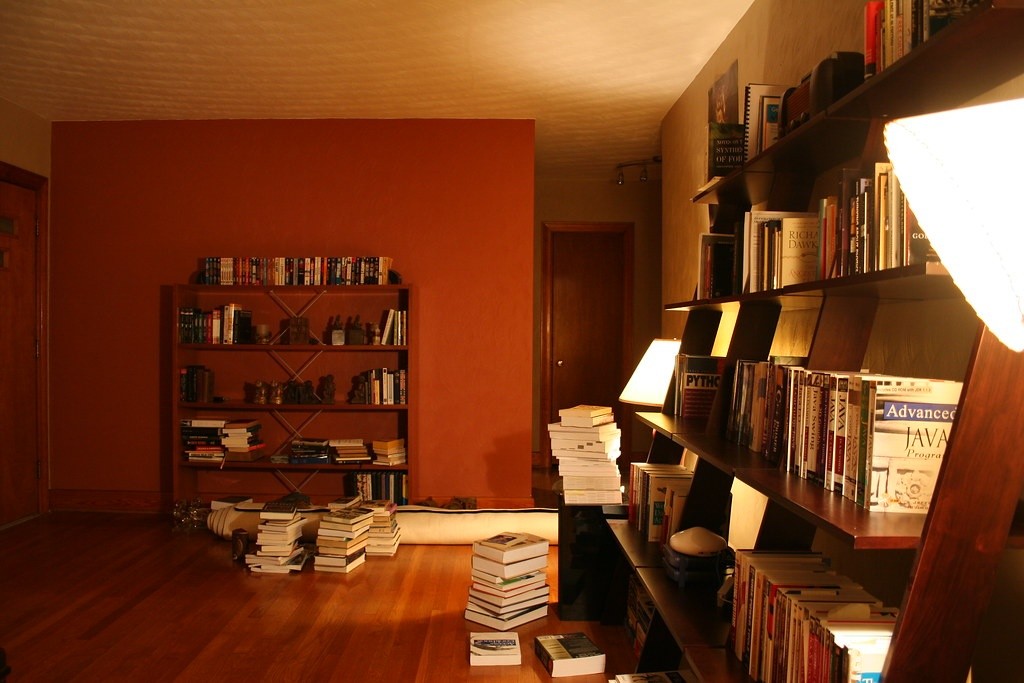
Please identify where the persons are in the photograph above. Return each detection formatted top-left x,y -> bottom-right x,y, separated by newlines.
714,92 -> 727,124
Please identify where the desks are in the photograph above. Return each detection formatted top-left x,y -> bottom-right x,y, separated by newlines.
552,469 -> 629,623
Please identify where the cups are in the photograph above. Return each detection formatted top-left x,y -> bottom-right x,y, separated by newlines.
231,529 -> 249,562
255,324 -> 269,344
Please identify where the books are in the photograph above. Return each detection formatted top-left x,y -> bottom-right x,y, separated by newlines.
179,255 -> 408,573
466,0 -> 963,683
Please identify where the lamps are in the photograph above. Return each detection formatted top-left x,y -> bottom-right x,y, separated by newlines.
619,337 -> 682,409
883,98 -> 1024,354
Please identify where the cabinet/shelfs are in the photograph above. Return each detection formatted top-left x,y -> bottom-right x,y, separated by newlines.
605,0 -> 1024,683
171,283 -> 419,511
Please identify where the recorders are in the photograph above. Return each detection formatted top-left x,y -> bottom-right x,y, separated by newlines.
777,51 -> 864,140
